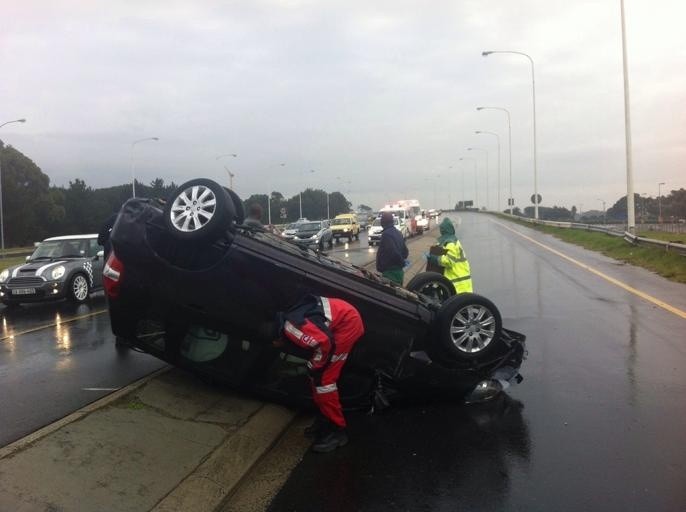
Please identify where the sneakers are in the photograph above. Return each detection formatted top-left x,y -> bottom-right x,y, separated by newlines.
303,416 -> 349,453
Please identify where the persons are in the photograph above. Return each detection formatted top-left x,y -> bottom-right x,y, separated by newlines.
377,213 -> 410,287
424,215 -> 474,295
259,294 -> 366,454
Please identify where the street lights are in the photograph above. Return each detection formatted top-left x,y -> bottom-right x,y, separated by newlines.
0,118 -> 25,131
266,163 -> 284,227
130,137 -> 158,197
451,50 -> 539,217
642,182 -> 665,221
598,198 -> 606,222
299,169 -> 315,220
215,153 -> 237,182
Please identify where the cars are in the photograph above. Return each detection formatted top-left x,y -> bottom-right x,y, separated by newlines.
2,233 -> 110,309
104,177 -> 526,419
281,200 -> 442,251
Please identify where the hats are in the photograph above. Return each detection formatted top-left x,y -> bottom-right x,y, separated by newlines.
439,217 -> 455,236
380,212 -> 394,227
256,319 -> 277,351
